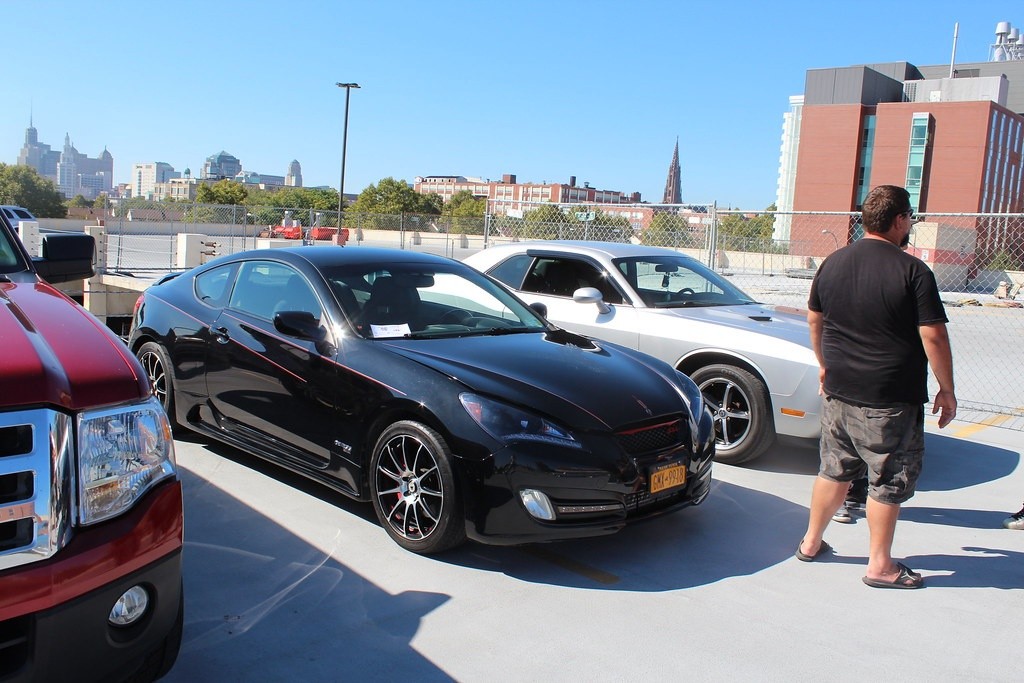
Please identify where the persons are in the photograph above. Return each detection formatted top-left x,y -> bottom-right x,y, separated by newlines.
830,465 -> 869,522
1003,507 -> 1024,531
796,185 -> 957,587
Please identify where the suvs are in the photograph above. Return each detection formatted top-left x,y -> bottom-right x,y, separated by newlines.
0,203 -> 188,683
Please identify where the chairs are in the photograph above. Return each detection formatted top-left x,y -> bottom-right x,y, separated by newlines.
533,260 -> 579,300
367,276 -> 424,340
287,274 -> 374,343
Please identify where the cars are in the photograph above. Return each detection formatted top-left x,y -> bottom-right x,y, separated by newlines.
370,238 -> 847,465
0,206 -> 38,231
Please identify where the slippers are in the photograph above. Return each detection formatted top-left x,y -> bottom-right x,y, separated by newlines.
795,539 -> 829,562
862,561 -> 924,589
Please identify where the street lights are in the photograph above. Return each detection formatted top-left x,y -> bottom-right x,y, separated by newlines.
334,82 -> 362,235
822,230 -> 839,253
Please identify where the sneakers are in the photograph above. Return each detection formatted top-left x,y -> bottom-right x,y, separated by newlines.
832,504 -> 851,522
1003,508 -> 1024,530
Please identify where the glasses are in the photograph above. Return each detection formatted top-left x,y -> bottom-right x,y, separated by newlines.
903,209 -> 914,216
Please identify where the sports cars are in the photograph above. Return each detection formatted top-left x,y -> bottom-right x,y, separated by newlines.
124,242 -> 721,556
